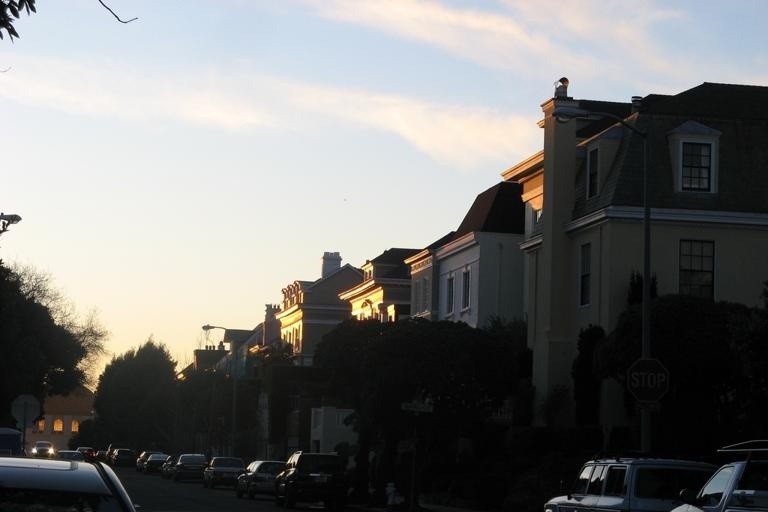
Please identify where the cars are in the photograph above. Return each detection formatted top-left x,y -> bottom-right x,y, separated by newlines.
200,455 -> 246,488
234,459 -> 286,500
0,456 -> 144,511
172,453 -> 210,482
31,440 -> 180,479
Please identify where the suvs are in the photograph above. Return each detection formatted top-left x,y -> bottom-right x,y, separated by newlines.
669,439 -> 767,512
272,451 -> 350,511
544,450 -> 720,511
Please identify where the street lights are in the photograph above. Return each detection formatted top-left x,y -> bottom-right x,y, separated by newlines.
203,326 -> 237,457
551,108 -> 653,456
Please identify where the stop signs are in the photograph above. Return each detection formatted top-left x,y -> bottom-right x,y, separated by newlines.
626,358 -> 671,404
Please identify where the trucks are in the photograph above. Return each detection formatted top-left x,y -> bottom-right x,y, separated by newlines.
0,428 -> 25,457
